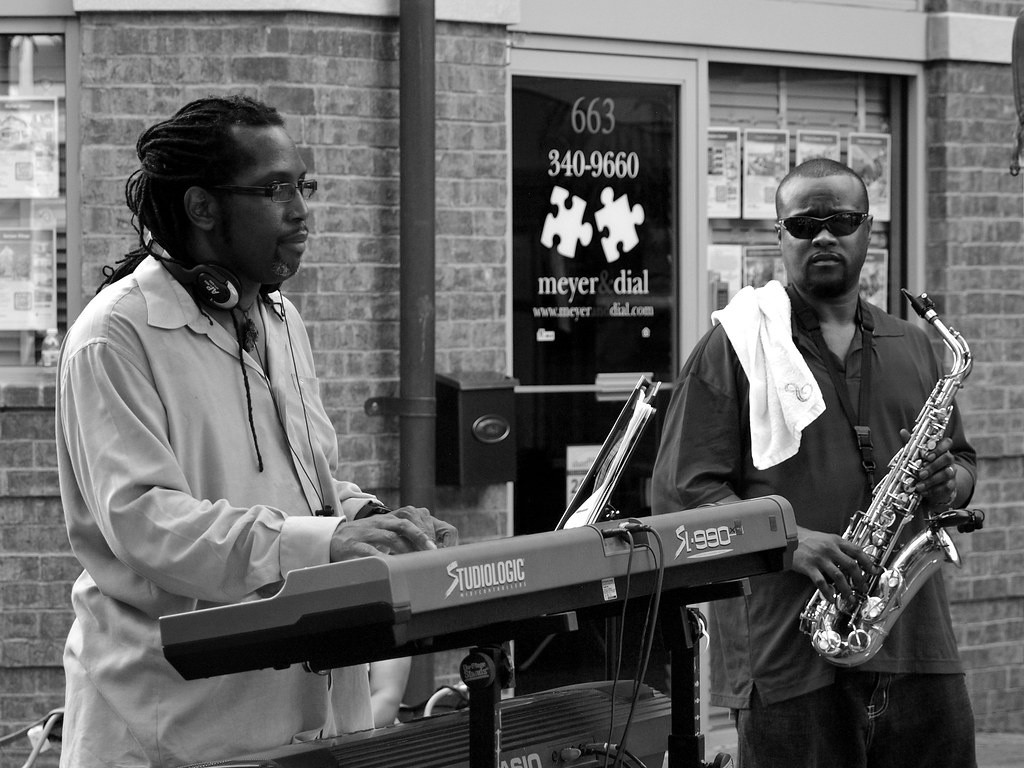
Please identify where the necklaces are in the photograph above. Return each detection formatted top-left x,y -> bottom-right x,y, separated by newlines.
235,302 -> 259,353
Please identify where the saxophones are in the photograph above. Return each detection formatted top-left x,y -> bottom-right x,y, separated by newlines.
800,288 -> 986,670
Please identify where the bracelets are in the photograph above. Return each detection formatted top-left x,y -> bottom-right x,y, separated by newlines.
936,487 -> 958,506
355,502 -> 392,520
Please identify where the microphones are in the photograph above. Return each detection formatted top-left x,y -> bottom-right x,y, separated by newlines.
958,517 -> 984,534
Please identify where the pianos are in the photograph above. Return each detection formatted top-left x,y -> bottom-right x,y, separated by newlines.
158,492 -> 800,683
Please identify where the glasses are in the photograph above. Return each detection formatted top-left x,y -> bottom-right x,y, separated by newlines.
778,211 -> 868,240
212,179 -> 318,204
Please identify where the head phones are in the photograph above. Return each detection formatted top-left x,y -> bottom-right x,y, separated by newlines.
147,232 -> 284,310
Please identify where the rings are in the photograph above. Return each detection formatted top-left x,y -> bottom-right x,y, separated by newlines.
947,466 -> 956,479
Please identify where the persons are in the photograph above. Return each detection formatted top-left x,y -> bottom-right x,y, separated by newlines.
55,95 -> 461,768
649,159 -> 979,768
